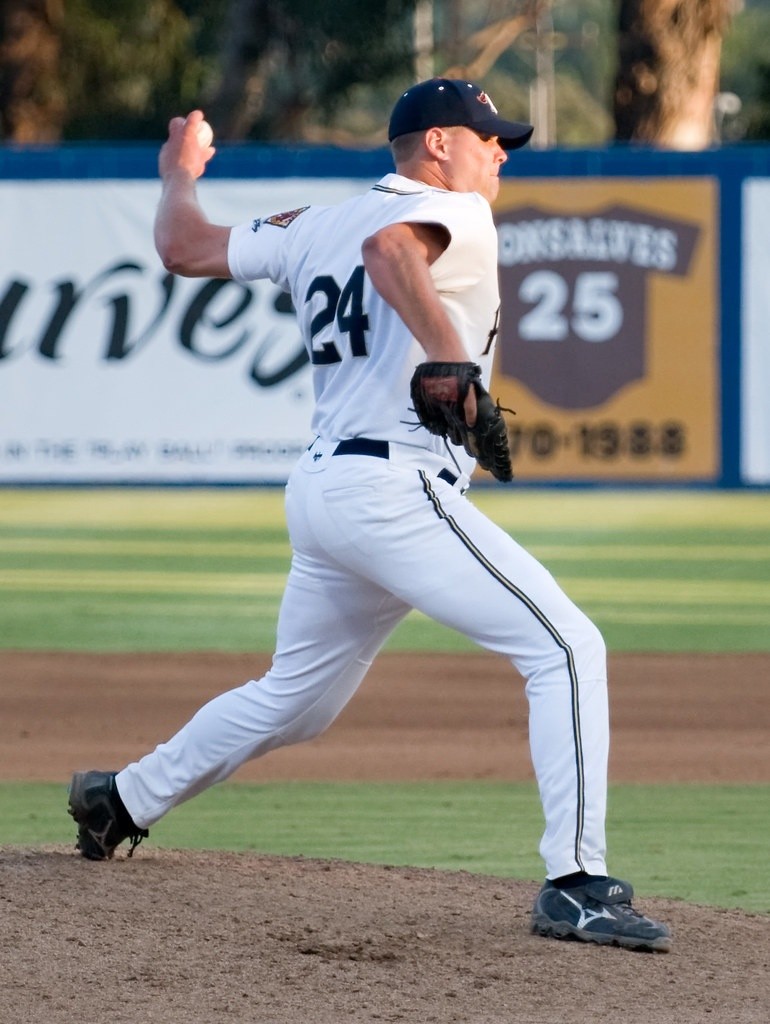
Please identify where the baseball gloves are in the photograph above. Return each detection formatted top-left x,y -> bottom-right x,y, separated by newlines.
409,361 -> 514,483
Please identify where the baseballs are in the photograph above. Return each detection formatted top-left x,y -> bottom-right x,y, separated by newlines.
184,119 -> 214,148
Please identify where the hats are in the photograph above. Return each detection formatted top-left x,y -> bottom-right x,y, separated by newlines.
389,76 -> 534,151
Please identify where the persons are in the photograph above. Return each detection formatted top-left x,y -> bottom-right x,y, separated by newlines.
68,79 -> 678,949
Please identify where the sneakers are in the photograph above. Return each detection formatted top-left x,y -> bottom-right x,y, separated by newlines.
66,770 -> 149,860
529,877 -> 672,952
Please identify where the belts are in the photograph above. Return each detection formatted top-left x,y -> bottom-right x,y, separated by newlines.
307,436 -> 465,494
